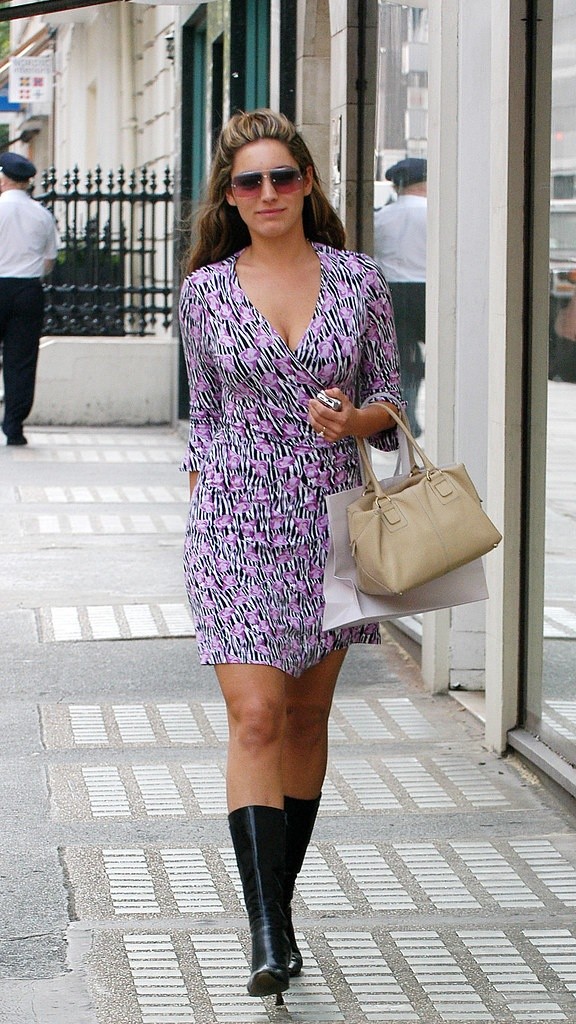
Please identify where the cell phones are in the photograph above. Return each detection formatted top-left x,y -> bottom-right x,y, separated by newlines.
317,393 -> 340,413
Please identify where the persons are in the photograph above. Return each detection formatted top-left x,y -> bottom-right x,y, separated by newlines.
373,158 -> 428,439
0,152 -> 61,445
162,110 -> 408,1006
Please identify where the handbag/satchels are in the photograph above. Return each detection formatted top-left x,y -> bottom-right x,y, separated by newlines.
347,401 -> 504,599
321,392 -> 502,635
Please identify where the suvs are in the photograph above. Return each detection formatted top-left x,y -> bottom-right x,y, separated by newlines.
549,200 -> 576,379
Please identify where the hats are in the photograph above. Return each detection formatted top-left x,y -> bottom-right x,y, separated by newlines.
384,157 -> 427,189
1,152 -> 37,182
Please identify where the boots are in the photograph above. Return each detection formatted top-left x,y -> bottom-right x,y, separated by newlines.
285,792 -> 325,977
228,805 -> 289,1006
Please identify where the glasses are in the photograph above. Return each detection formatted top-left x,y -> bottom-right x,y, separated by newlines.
229,166 -> 306,199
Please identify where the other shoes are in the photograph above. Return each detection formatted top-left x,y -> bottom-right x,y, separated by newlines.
8,435 -> 27,445
